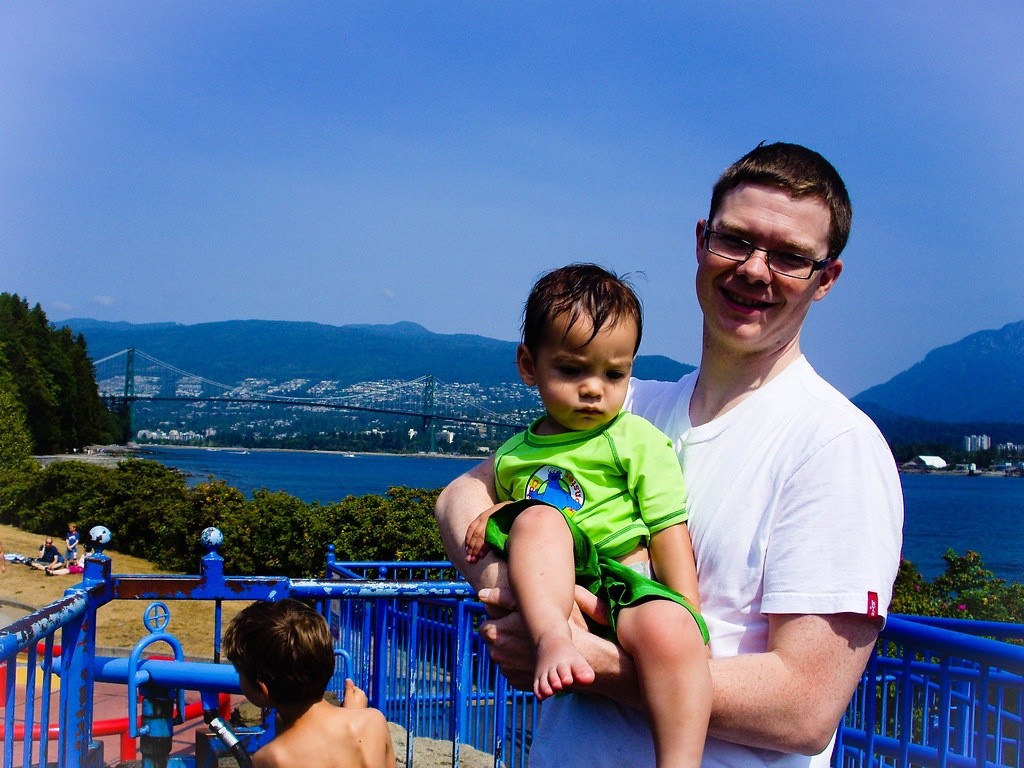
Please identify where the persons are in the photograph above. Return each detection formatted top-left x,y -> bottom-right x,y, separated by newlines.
462,260 -> 715,768
431,140 -> 907,768
0,542 -> 6,574
64,522 -> 80,568
45,543 -> 93,576
222,598 -> 398,768
30,538 -> 64,570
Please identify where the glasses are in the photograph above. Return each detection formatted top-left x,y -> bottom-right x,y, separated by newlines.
45,542 -> 52,544
705,218 -> 831,280
